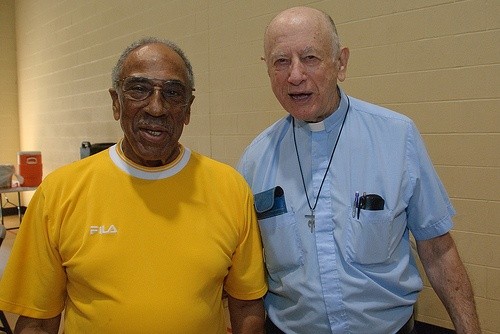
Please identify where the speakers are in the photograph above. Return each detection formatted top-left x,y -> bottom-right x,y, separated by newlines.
19,150 -> 42,187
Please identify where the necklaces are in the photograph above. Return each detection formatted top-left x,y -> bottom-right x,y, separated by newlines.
292,92 -> 350,233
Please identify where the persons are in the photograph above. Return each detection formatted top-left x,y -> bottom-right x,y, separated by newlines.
239,7 -> 483,334
2,36 -> 271,334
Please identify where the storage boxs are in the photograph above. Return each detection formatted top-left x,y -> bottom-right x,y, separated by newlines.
18,151 -> 42,187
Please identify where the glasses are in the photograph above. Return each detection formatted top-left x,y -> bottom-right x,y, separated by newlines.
114,76 -> 194,109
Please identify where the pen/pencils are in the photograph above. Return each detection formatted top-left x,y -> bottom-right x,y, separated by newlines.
351,192 -> 359,219
357,193 -> 368,218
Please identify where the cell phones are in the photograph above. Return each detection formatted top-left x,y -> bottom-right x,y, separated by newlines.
361,194 -> 385,212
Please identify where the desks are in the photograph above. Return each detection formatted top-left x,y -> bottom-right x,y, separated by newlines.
1,186 -> 34,228
